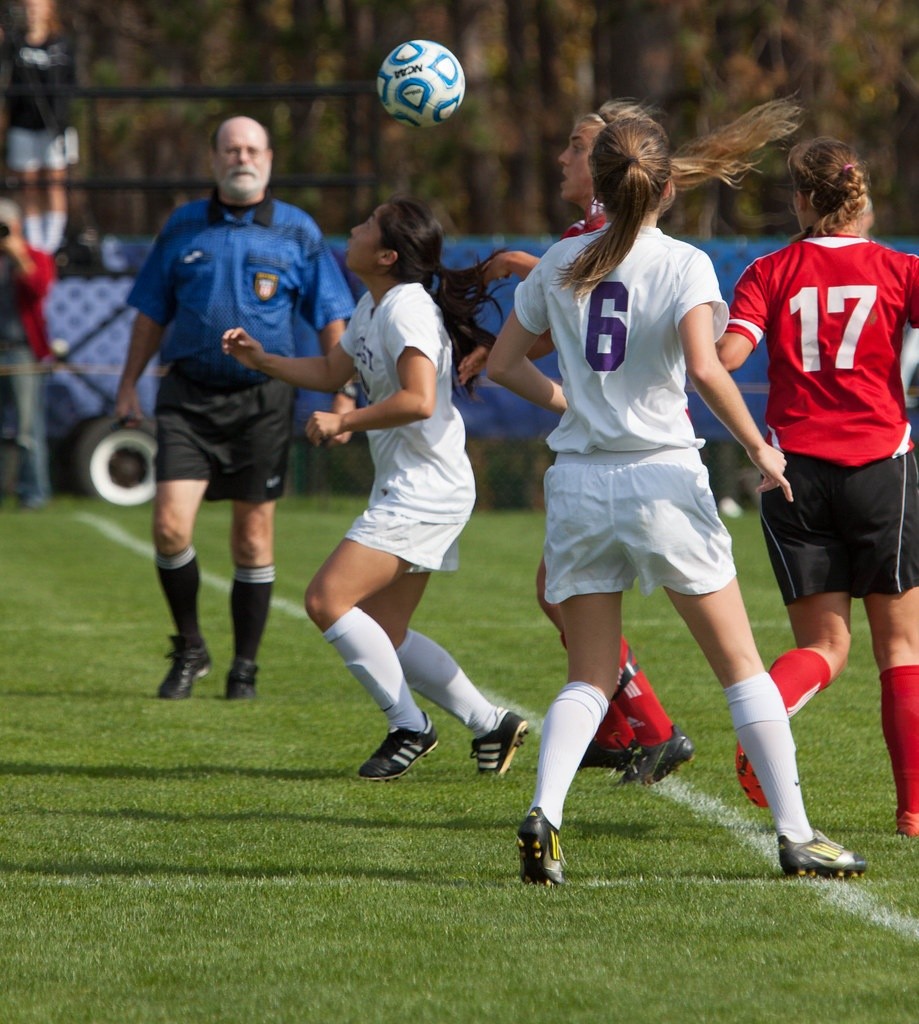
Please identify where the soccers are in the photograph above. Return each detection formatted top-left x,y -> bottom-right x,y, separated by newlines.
371,34 -> 471,133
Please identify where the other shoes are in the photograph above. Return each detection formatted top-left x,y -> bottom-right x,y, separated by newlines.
735,741 -> 770,809
895,808 -> 919,838
14,492 -> 51,514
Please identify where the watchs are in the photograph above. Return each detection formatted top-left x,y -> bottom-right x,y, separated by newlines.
333,383 -> 357,399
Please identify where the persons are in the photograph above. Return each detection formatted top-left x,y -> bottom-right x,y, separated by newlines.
485,104 -> 689,786
0,0 -> 80,509
488,122 -> 869,882
221,199 -> 525,781
114,116 -> 356,702
711,139 -> 919,839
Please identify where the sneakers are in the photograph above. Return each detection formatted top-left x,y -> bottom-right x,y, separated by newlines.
615,725 -> 695,787
470,705 -> 530,780
358,711 -> 438,781
515,806 -> 567,883
157,633 -> 212,699
576,741 -> 637,774
778,830 -> 868,880
226,658 -> 258,698
